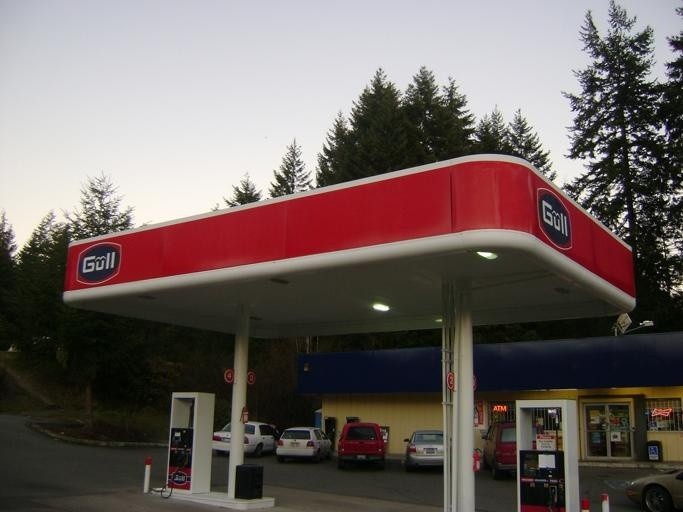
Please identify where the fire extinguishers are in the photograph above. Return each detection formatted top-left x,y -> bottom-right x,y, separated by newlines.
239,406 -> 248,424
474,448 -> 482,471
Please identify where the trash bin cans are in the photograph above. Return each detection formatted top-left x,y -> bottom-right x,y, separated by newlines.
646,440 -> 662,462
235,464 -> 264,500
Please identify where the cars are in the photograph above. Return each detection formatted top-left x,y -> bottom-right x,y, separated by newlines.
275,425 -> 332,462
620,468 -> 683,511
7,335 -> 57,354
208,420 -> 280,457
403,430 -> 443,471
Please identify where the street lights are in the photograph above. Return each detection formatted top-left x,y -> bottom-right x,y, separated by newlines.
613,320 -> 653,337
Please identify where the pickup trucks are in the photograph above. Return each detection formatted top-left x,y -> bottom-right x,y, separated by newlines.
335,422 -> 384,470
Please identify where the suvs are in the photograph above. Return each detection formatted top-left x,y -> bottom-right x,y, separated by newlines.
480,420 -> 545,479
